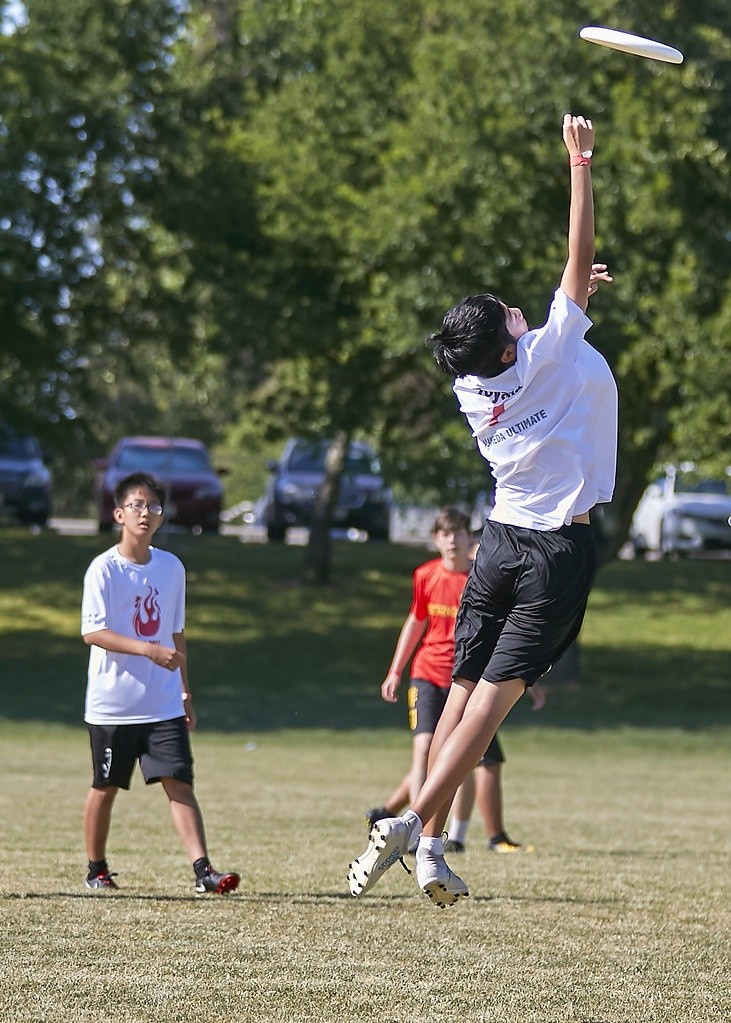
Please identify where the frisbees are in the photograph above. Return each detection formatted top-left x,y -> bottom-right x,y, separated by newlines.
579,26 -> 684,64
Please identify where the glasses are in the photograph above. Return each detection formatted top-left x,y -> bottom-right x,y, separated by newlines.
121,501 -> 165,515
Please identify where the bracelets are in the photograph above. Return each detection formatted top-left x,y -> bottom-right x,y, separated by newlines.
570,150 -> 593,167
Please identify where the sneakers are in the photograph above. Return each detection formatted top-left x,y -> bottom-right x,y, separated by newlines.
415,831 -> 469,909
345,812 -> 418,898
84,870 -> 120,890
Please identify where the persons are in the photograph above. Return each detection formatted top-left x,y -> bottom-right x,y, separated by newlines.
348,114 -> 618,908
80,472 -> 240,895
366,509 -> 475,852
443,680 -> 545,852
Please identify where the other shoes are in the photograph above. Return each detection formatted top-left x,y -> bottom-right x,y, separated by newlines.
444,839 -> 466,853
490,842 -> 535,853
408,838 -> 420,856
365,806 -> 395,831
194,865 -> 240,894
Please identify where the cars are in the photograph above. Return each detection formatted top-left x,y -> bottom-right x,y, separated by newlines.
264,435 -> 393,543
617,466 -> 731,563
1,432 -> 57,529
93,434 -> 228,533
